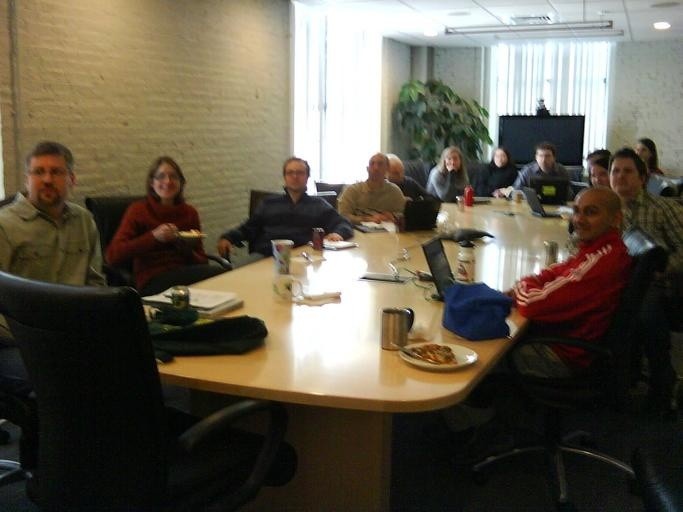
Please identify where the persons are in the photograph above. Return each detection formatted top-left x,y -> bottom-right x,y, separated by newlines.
585,148 -> 608,164
0,141 -> 108,504
588,157 -> 609,187
475,149 -> 518,197
609,150 -> 683,407
426,147 -> 469,202
336,153 -> 406,222
440,185 -> 631,478
380,154 -> 435,204
218,158 -> 354,259
635,138 -> 664,175
101,155 -> 224,294
505,142 -> 574,203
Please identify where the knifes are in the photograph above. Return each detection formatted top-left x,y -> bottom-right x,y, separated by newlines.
389,341 -> 441,364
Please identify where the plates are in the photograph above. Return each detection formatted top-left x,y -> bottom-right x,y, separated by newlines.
175,234 -> 206,240
399,342 -> 480,372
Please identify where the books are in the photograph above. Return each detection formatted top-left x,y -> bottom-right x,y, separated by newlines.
142,298 -> 243,319
140,284 -> 236,310
304,237 -> 357,252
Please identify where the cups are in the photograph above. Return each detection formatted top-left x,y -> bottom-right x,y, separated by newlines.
271,238 -> 293,275
380,307 -> 414,351
512,191 -> 523,203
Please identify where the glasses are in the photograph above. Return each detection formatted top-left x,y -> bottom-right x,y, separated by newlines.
27,166 -> 71,176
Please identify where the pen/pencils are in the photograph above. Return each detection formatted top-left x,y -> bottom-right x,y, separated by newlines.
163,293 -> 173,300
403,268 -> 417,276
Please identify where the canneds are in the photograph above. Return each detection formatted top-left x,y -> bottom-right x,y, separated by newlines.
544,240 -> 558,266
172,286 -> 190,310
313,229 -> 324,250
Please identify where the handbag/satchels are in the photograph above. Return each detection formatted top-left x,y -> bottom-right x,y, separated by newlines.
440,277 -> 513,343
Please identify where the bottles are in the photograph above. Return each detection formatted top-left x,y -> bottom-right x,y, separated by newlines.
171,285 -> 189,308
543,240 -> 559,267
455,195 -> 463,211
313,227 -> 324,250
463,184 -> 473,205
456,240 -> 475,285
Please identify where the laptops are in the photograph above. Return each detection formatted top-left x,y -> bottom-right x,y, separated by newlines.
530,175 -> 569,206
420,235 -> 457,302
404,199 -> 442,230
520,184 -> 563,218
